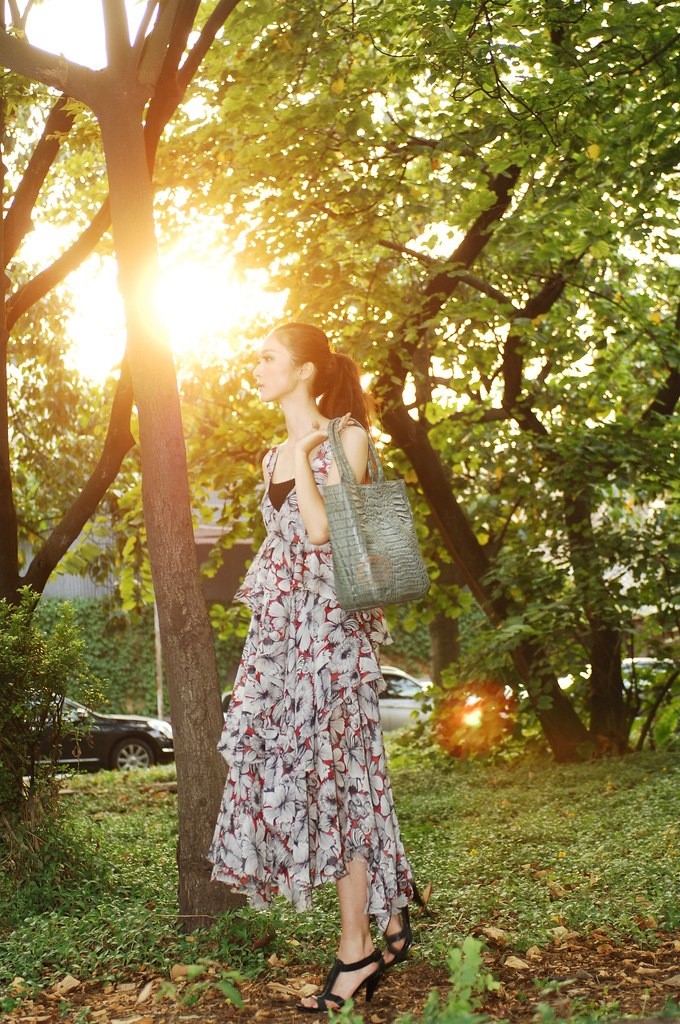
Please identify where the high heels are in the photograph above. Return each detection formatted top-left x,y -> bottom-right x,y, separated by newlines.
297,949 -> 385,1010
382,879 -> 424,963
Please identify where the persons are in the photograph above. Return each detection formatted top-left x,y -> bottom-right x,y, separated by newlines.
206,327 -> 429,1016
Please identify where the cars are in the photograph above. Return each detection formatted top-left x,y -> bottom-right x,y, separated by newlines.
24,686 -> 174,774
378,667 -> 436,730
519,656 -> 675,718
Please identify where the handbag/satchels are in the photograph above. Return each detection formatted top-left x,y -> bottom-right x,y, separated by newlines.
321,417 -> 431,612
